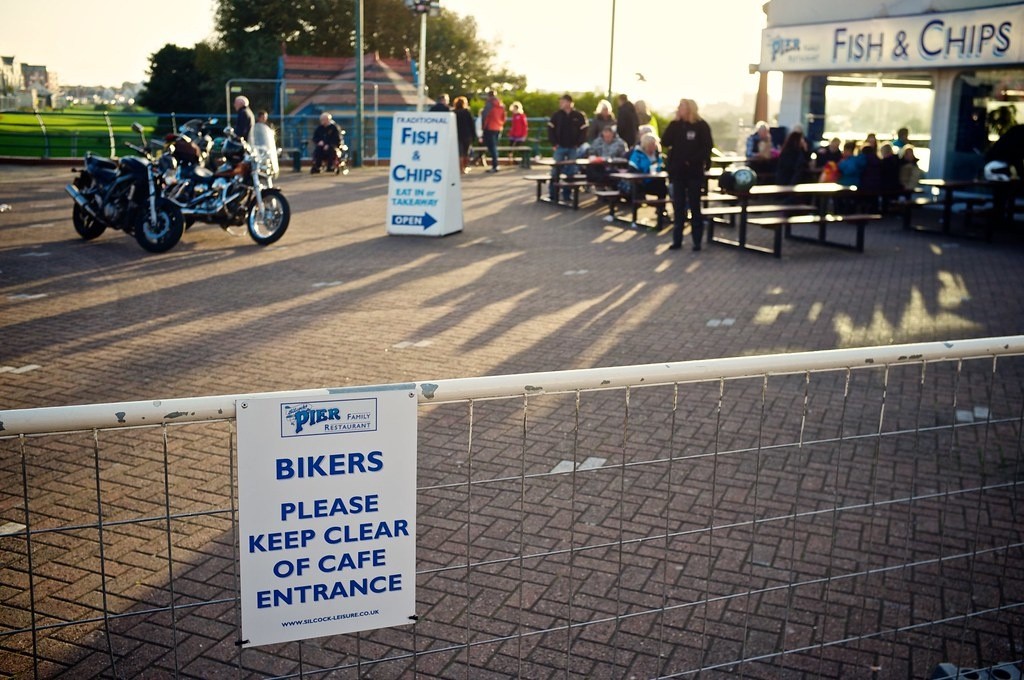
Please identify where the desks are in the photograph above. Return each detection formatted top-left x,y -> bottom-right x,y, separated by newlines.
917,175 -> 1024,231
530,156 -> 628,205
707,182 -> 856,257
608,167 -> 725,231
711,155 -> 750,165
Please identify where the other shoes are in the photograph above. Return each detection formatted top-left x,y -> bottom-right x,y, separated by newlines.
669,244 -> 682,249
325,168 -> 334,172
462,167 -> 471,174
310,167 -> 320,174
486,166 -> 499,172
691,247 -> 701,252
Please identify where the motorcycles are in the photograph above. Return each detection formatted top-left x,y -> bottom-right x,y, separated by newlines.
65,112 -> 291,254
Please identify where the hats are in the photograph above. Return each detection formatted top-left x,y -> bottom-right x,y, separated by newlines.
557,94 -> 572,102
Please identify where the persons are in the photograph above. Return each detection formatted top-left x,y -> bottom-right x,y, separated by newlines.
661,100 -> 713,251
548,95 -> 668,217
235,96 -> 278,176
746,122 -> 919,218
476,91 -> 507,172
429,94 -> 450,112
310,113 -> 342,173
986,123 -> 1024,236
452,96 -> 478,174
508,101 -> 528,165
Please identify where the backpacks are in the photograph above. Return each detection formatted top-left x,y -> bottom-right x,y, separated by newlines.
819,162 -> 839,183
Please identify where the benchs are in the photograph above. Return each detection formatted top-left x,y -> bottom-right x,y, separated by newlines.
889,197 -> 1024,241
747,212 -> 881,258
633,193 -> 737,231
524,173 -> 588,200
552,182 -> 599,210
470,145 -> 531,168
702,205 -> 818,237
596,190 -> 619,218
